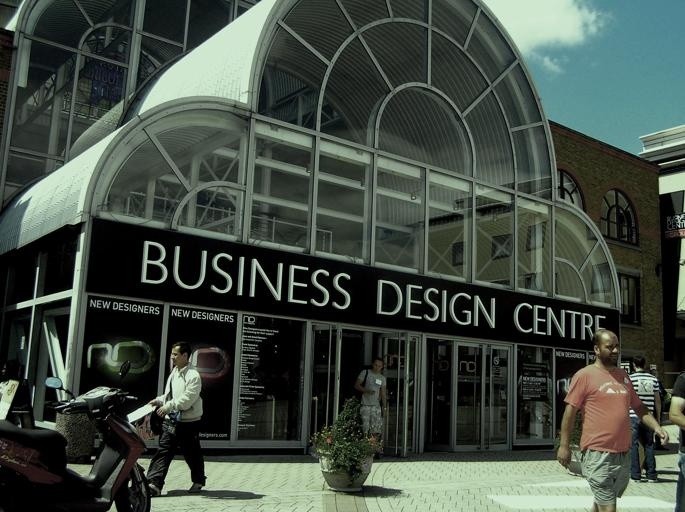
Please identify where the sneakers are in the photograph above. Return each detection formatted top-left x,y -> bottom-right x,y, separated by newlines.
188,482 -> 203,494
149,483 -> 160,496
648,478 -> 658,483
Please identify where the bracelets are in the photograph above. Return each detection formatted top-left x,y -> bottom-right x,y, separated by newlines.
384,408 -> 387,410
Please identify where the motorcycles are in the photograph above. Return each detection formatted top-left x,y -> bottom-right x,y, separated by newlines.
1,361 -> 150,511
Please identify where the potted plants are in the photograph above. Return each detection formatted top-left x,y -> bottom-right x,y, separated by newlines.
553,412 -> 585,478
308,397 -> 385,492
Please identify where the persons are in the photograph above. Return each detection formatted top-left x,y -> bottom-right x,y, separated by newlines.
356,358 -> 387,459
557,329 -> 667,512
669,372 -> 685,512
648,368 -> 668,450
145,341 -> 205,496
628,356 -> 662,483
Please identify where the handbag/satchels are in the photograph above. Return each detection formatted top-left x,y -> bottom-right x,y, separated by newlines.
161,411 -> 181,435
355,383 -> 365,403
